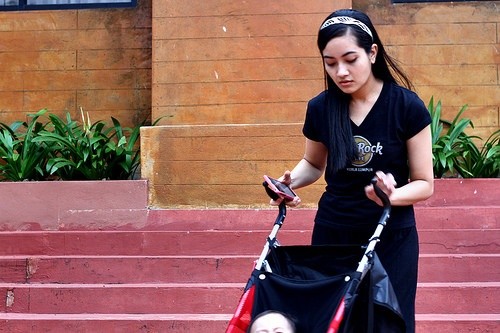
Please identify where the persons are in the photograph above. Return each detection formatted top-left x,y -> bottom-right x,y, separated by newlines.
269,9 -> 434,333
247,310 -> 301,333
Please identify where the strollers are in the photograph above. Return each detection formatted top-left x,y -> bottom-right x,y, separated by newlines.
225,180 -> 408,333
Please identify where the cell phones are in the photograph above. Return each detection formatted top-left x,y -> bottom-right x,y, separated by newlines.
264,175 -> 301,202
